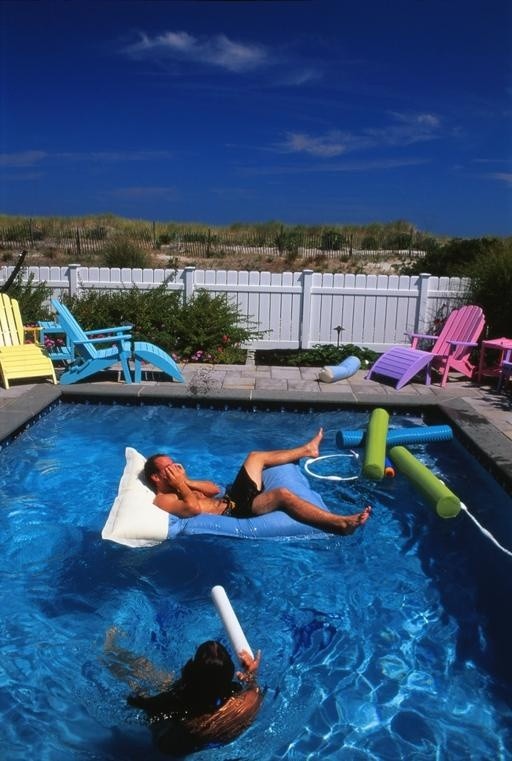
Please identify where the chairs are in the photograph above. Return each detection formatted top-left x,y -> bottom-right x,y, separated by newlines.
50,298 -> 184,386
0,293 -> 58,389
365,305 -> 486,390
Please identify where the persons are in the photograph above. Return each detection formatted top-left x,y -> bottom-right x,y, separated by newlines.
94,623 -> 264,757
145,425 -> 372,538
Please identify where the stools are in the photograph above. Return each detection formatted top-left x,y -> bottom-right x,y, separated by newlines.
37,321 -> 74,368
478,336 -> 512,393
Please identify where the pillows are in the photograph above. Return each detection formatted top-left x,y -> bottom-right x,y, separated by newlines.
318,356 -> 362,382
